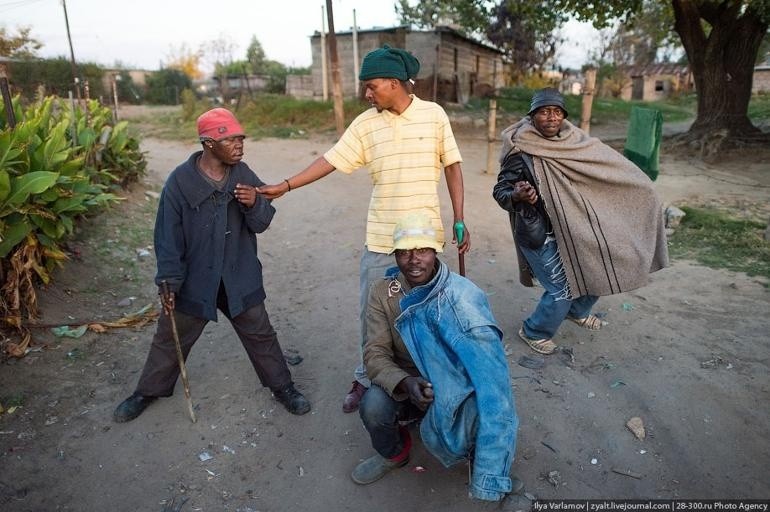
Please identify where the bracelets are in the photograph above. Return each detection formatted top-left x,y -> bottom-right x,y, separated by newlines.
283,178 -> 291,194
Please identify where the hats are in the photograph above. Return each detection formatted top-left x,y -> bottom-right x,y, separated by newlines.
388,215 -> 444,254
526,87 -> 569,118
359,44 -> 420,81
196,107 -> 248,142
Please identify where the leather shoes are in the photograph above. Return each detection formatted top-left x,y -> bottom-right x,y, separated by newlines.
112,390 -> 161,422
273,383 -> 312,415
343,380 -> 367,412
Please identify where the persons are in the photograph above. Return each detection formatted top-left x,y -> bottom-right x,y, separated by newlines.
348,217 -> 515,502
491,89 -> 672,355
107,105 -> 309,426
255,44 -> 472,415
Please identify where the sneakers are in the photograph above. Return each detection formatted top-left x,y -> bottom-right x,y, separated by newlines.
506,471 -> 526,496
350,455 -> 410,484
565,313 -> 602,330
518,323 -> 560,355
